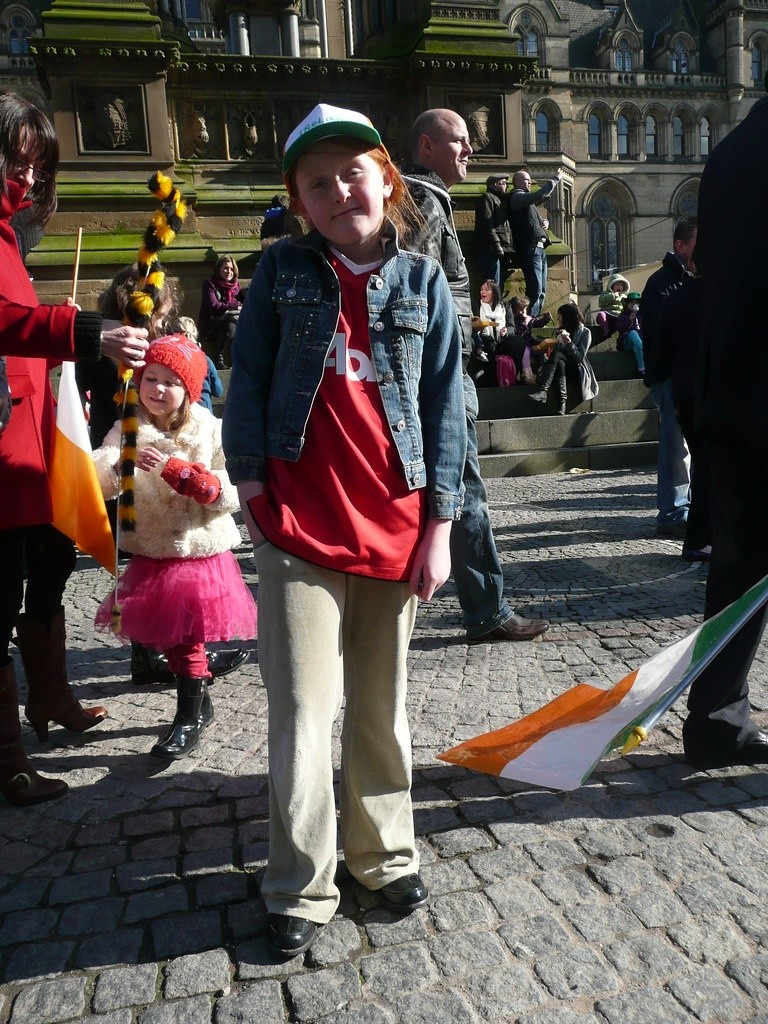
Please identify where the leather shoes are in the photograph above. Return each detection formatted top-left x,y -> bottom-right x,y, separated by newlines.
130,659 -> 178,686
208,649 -> 250,676
344,866 -> 428,910
267,914 -> 316,956
467,614 -> 551,644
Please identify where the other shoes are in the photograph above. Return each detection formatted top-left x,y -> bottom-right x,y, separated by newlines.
682,545 -> 712,560
684,714 -> 768,760
641,371 -> 646,377
658,508 -> 690,537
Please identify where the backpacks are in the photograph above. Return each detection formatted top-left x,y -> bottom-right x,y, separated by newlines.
496,355 -> 517,386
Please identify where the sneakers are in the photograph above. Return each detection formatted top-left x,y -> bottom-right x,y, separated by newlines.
476,351 -> 489,361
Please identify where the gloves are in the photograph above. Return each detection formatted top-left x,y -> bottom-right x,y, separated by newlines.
160,457 -> 221,506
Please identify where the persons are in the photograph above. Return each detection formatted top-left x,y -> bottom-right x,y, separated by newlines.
470,278 -> 553,387
0,91 -> 148,810
61,260 -> 249,685
507,166 -> 564,313
640,72 -> 768,768
222,103 -> 466,956
259,194 -> 303,239
474,175 -> 514,296
528,304 -> 598,415
198,254 -> 247,368
618,292 -> 646,376
385,109 -> 549,642
597,273 -> 631,340
89,332 -> 260,759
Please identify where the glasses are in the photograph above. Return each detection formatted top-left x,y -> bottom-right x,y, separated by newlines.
499,182 -> 506,186
9,157 -> 51,183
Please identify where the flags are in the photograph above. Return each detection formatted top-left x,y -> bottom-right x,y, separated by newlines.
436,565 -> 768,792
47,360 -> 120,576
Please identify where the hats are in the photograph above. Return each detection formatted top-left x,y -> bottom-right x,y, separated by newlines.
144,333 -> 208,404
621,293 -> 641,304
486,175 -> 509,186
283,103 -> 381,173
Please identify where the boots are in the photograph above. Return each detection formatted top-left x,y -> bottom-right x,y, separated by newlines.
527,369 -> 553,403
552,376 -> 567,414
16,606 -> 108,741
522,367 -> 536,384
215,334 -> 232,369
150,679 -> 206,759
0,656 -> 69,803
197,688 -> 214,736
